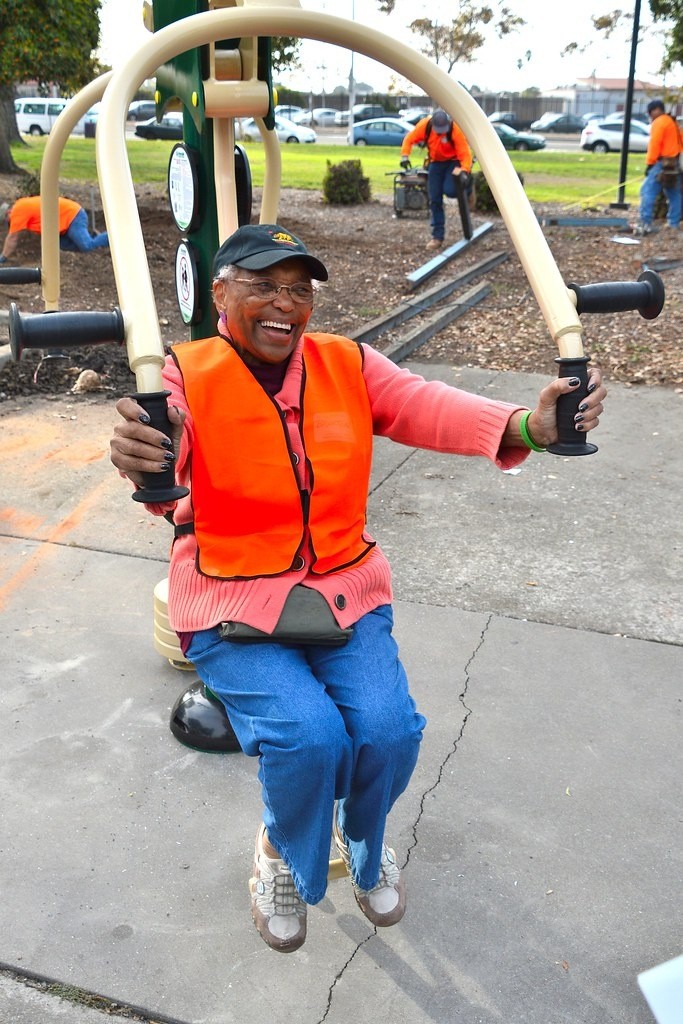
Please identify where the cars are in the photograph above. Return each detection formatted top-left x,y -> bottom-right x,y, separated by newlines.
235,105 -> 339,144
491,122 -> 547,151
335,104 -> 430,146
128,100 -> 183,140
531,110 -> 649,133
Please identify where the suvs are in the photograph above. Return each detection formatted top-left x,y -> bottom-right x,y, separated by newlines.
580,119 -> 650,153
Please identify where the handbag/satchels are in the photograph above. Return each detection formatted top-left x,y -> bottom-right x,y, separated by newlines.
218,579 -> 353,645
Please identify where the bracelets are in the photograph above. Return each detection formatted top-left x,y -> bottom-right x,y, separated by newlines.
518,411 -> 548,452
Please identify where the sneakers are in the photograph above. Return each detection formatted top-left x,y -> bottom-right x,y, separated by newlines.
249,822 -> 306,952
330,794 -> 406,927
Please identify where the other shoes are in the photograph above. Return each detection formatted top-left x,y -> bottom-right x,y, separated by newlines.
468,183 -> 476,208
629,221 -> 650,232
425,237 -> 442,250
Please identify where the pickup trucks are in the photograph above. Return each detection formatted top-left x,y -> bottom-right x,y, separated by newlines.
488,111 -> 536,131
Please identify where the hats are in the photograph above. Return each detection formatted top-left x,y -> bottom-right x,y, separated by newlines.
0,203 -> 10,225
432,110 -> 448,133
213,223 -> 328,283
647,99 -> 663,114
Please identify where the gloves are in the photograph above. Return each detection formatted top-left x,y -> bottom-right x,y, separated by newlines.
644,164 -> 653,176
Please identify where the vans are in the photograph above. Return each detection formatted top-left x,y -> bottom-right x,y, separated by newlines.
14,97 -> 99,136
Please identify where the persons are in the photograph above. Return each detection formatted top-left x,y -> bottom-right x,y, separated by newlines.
111,224 -> 607,954
639,99 -> 683,231
0,195 -> 110,262
400,109 -> 473,250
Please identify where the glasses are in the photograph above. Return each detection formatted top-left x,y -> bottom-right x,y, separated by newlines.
224,275 -> 319,303
